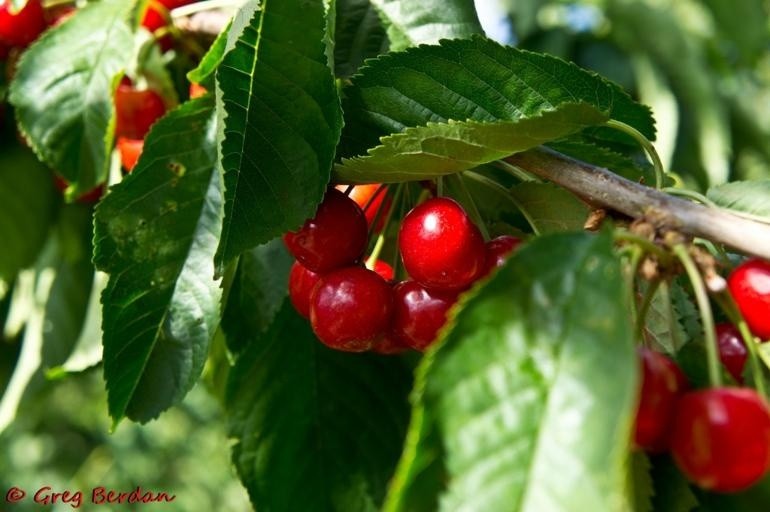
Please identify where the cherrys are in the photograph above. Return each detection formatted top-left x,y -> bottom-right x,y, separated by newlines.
0,0 -> 207,201
280,177 -> 525,354
624,221 -> 770,491
